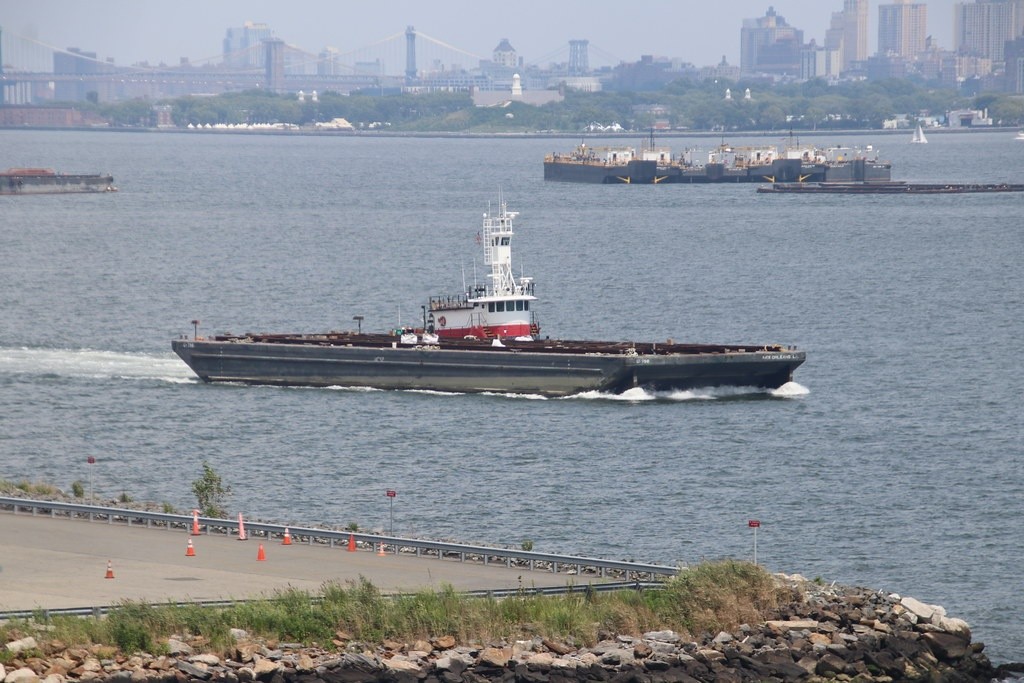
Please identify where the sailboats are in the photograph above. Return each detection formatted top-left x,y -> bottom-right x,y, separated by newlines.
909,126 -> 928,143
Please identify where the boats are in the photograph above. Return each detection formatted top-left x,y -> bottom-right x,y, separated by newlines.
0,168 -> 116,194
171,188 -> 807,397
543,125 -> 892,185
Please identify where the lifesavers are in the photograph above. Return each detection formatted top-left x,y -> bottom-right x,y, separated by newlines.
439,316 -> 446,327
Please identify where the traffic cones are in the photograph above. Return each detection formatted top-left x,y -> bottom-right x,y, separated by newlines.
104,559 -> 114,578
377,541 -> 384,555
346,534 -> 357,552
282,526 -> 293,545
236,512 -> 249,540
256,544 -> 266,561
185,538 -> 196,556
191,511 -> 201,535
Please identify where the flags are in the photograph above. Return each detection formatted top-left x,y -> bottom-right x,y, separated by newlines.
475,231 -> 481,245
